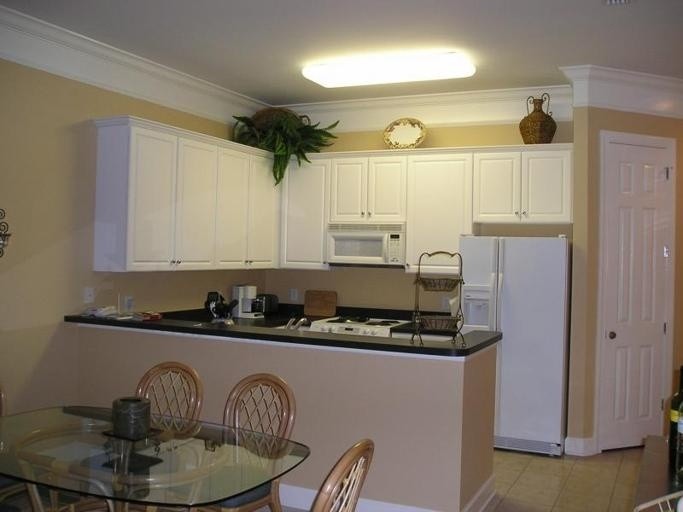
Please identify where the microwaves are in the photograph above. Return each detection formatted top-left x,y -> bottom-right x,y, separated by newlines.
325,228 -> 404,267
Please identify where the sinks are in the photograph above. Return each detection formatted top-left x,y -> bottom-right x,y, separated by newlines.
273,324 -> 310,331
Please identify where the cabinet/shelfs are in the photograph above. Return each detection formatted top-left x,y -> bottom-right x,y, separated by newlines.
471,142 -> 574,223
405,147 -> 474,276
214,134 -> 282,272
334,148 -> 407,228
282,151 -> 332,270
90,116 -> 215,275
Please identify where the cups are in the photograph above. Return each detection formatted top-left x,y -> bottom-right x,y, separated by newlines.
111,395 -> 150,441
116,292 -> 133,320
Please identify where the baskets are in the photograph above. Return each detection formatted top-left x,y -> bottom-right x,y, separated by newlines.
421,315 -> 461,330
421,278 -> 461,291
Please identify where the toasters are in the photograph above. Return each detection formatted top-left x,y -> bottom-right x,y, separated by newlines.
254,293 -> 279,315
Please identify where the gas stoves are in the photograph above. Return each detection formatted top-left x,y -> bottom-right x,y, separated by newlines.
310,311 -> 409,337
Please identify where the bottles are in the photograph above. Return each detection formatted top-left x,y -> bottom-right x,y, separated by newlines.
668,365 -> 683,489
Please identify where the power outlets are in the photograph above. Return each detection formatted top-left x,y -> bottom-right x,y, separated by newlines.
289,288 -> 298,302
82,286 -> 96,304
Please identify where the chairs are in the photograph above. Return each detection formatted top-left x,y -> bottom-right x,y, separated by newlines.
310,439 -> 375,512
81,362 -> 204,511
199,372 -> 296,512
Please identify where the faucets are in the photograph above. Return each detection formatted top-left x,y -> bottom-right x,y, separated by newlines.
285,317 -> 309,330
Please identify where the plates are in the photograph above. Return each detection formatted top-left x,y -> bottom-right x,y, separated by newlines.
382,116 -> 426,151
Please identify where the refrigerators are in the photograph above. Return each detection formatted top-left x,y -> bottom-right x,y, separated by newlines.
455,233 -> 567,457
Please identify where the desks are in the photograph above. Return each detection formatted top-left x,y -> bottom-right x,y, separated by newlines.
0,406 -> 311,512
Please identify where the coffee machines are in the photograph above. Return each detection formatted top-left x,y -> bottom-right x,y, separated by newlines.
230,284 -> 265,319
